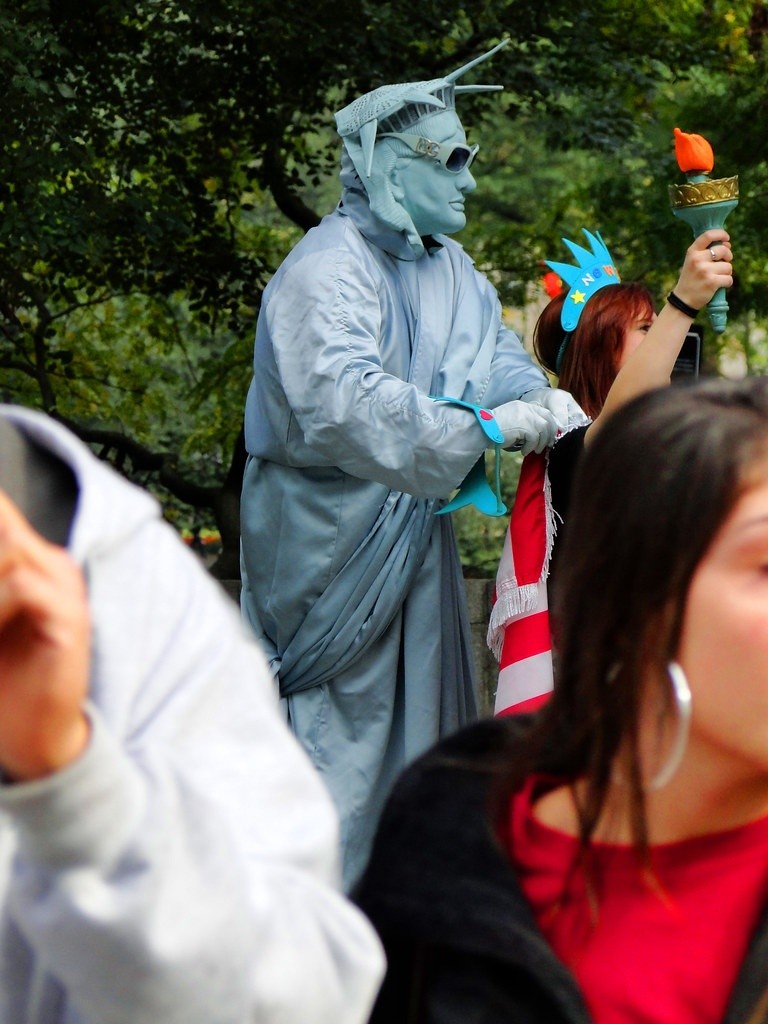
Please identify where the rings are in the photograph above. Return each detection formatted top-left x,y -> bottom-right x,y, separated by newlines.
707,244 -> 720,263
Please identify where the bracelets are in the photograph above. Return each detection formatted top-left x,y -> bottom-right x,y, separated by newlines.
667,291 -> 700,320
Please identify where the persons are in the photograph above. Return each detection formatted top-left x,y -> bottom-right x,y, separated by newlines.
239,39 -> 589,889
0,229 -> 768,1024
